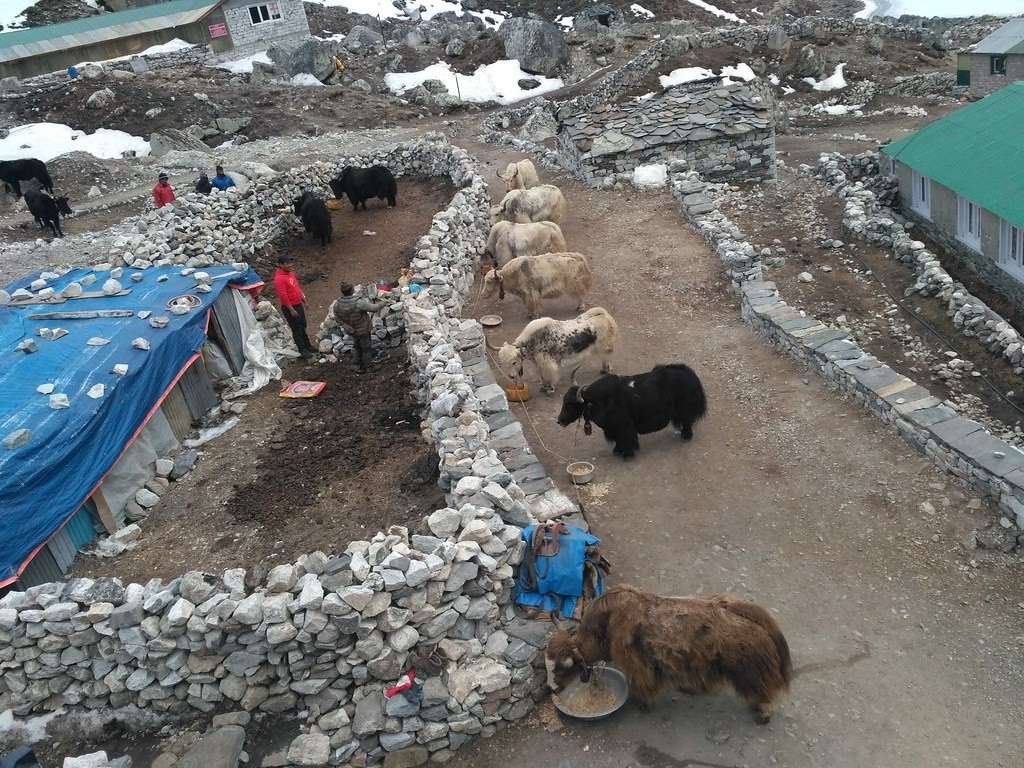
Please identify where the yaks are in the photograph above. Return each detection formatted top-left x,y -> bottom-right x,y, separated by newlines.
557,362 -> 708,462
292,192 -> 333,246
476,220 -> 566,270
496,159 -> 539,193
481,251 -> 592,320
488,184 -> 564,227
0,159 -> 53,197
329,165 -> 398,212
545,586 -> 792,725
24,187 -> 73,237
486,306 -> 621,396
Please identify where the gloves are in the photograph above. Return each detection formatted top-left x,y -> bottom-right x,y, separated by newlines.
345,324 -> 355,333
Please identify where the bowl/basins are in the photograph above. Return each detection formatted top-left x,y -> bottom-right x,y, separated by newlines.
327,200 -> 344,210
480,314 -> 502,326
567,461 -> 594,484
552,666 -> 630,721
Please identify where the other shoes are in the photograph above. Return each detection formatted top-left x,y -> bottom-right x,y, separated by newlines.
409,654 -> 442,675
367,365 -> 381,372
418,647 -> 448,670
351,365 -> 359,371
301,351 -> 313,358
307,345 -> 319,353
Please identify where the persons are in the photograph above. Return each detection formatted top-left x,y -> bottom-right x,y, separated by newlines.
153,171 -> 177,209
196,170 -> 213,194
212,165 -> 237,191
332,280 -> 389,374
272,253 -> 320,359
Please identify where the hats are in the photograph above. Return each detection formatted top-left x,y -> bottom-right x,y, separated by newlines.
277,254 -> 293,263
340,281 -> 353,293
200,171 -> 206,177
158,173 -> 168,180
